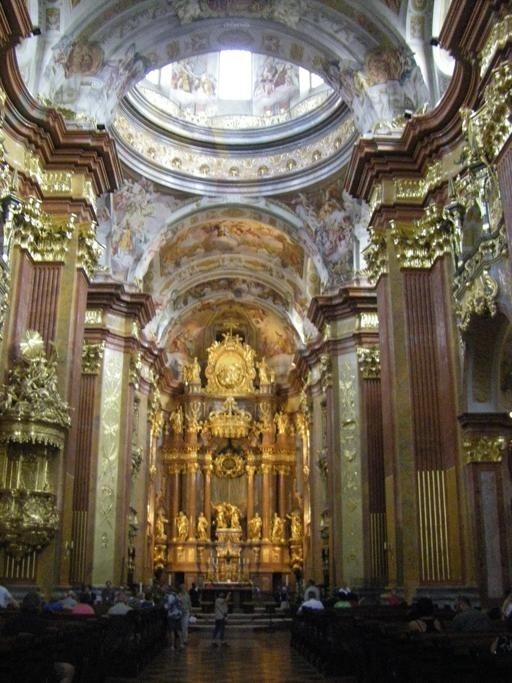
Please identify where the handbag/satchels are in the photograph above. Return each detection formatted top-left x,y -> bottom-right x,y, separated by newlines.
167,599 -> 183,620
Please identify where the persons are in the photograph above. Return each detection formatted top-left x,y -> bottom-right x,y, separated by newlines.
286,511 -> 301,539
269,512 -> 286,539
155,509 -> 169,539
184,354 -> 202,383
247,511 -> 262,541
171,54 -> 301,101
224,502 -> 242,528
211,590 -> 232,647
273,410 -> 288,433
190,580 -> 203,618
273,576 -> 511,683
176,511 -> 189,541
196,511 -> 209,541
0,574 -> 194,683
169,406 -> 184,434
255,354 -> 272,386
209,499 -> 228,528
185,402 -> 204,430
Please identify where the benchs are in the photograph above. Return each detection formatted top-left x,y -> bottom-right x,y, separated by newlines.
0,602 -> 171,683
290,605 -> 511,683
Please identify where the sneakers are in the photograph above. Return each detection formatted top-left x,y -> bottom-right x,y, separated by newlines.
211,642 -> 230,647
